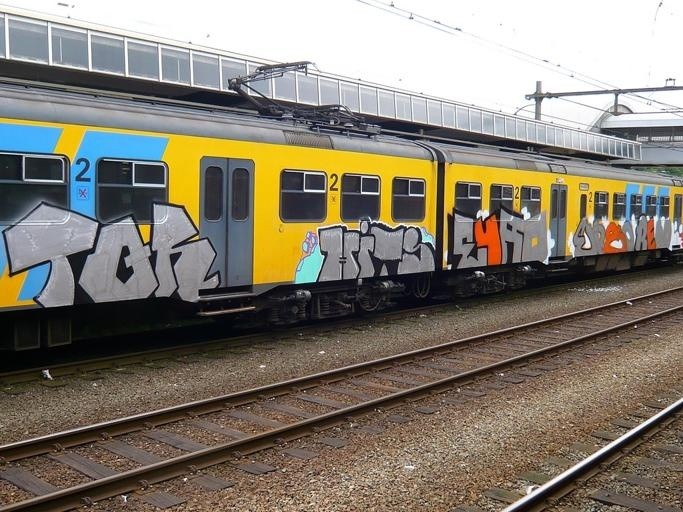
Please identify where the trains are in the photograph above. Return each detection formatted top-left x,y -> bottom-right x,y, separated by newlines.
0,77 -> 683,372
